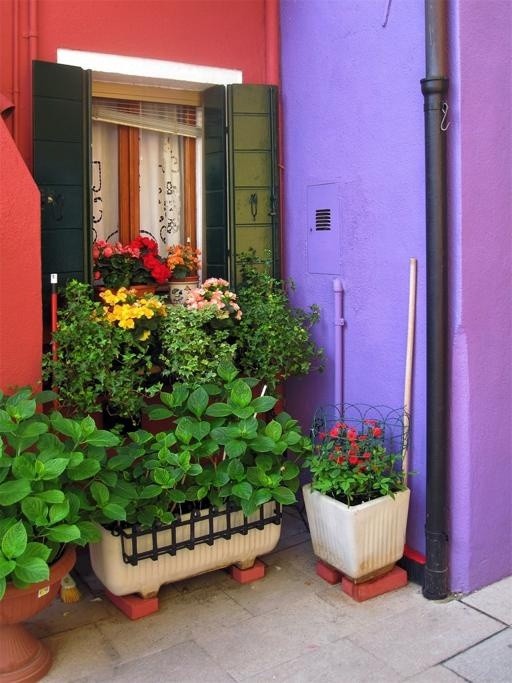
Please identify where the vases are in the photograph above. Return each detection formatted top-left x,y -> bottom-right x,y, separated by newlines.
167,275 -> 200,306
299,480 -> 413,581
96,282 -> 158,303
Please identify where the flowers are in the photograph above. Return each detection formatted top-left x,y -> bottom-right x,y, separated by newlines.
165,243 -> 203,273
301,417 -> 416,508
92,236 -> 172,287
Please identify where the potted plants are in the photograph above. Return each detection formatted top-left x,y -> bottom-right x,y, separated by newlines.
88,356 -> 316,600
0,381 -> 119,683
